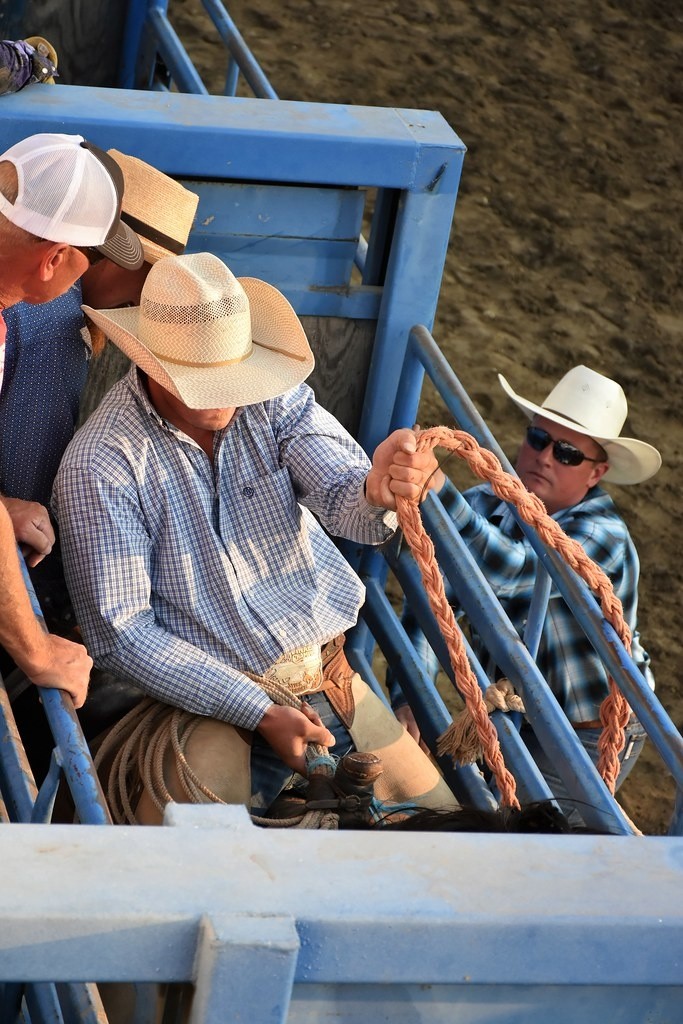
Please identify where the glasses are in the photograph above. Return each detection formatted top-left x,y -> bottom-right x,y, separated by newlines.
35,239 -> 104,267
522,426 -> 598,468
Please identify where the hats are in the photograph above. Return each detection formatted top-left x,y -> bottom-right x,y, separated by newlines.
0,133 -> 144,272
106,149 -> 200,266
81,251 -> 316,411
497,363 -> 663,490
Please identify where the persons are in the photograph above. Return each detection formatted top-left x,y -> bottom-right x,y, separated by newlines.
413,364 -> 662,827
50,251 -> 462,1024
0,37 -> 198,707
